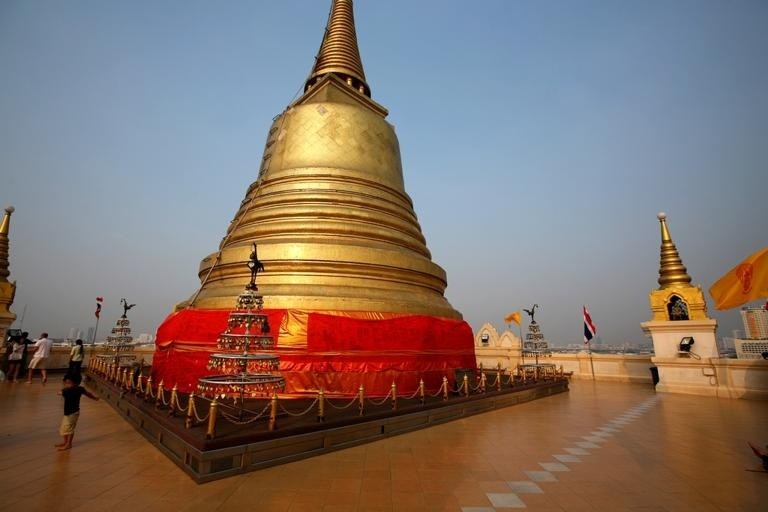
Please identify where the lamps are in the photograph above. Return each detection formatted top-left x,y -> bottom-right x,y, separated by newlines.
680,337 -> 694,351
481,334 -> 489,343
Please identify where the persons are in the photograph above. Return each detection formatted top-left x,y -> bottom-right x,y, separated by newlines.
54,372 -> 99,451
2,331 -> 53,385
747,441 -> 768,472
67,339 -> 85,374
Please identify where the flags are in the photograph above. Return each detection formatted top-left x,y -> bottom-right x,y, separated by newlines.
581,305 -> 597,344
708,247 -> 768,311
95,297 -> 104,319
504,312 -> 521,325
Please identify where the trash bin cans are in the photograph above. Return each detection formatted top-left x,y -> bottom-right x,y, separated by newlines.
650,367 -> 659,389
